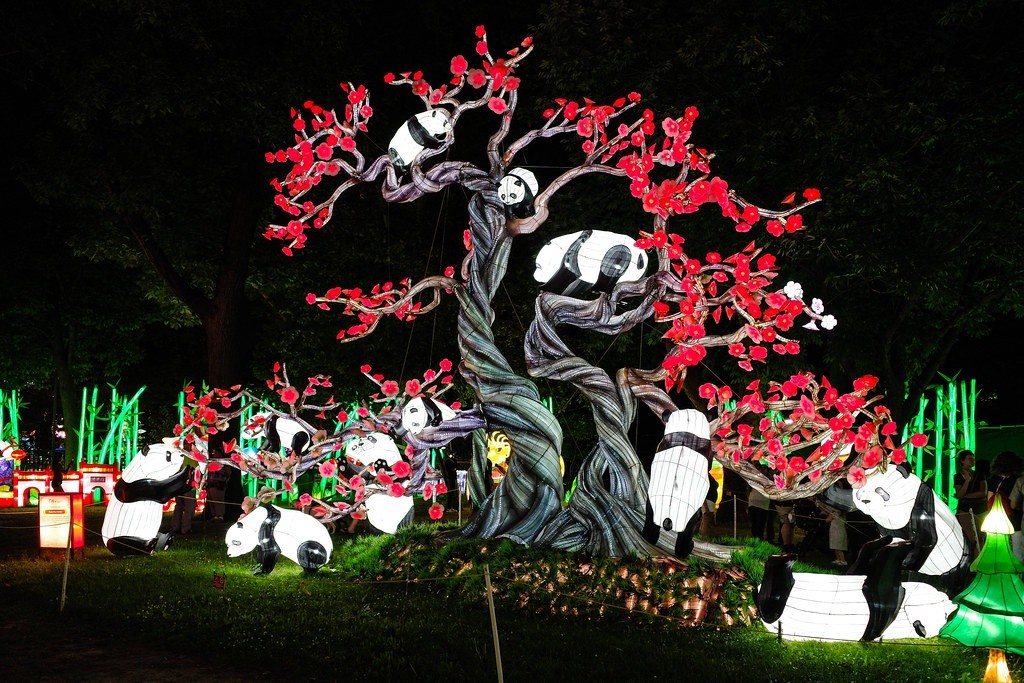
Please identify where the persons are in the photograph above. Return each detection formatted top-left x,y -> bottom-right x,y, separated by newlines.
170,438 -> 196,535
51,452 -> 73,493
694,480 -> 849,567
953,450 -> 1024,564
206,448 -> 231,522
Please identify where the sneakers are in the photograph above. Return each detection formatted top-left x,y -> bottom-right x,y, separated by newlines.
832,560 -> 841,563
838,561 -> 848,565
